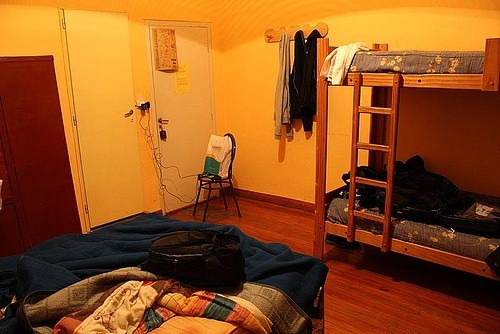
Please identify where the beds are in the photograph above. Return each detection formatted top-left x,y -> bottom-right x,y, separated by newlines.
314,37 -> 500,279
0,210 -> 328,334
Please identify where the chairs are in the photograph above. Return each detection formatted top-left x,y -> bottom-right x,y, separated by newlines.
192,132 -> 242,224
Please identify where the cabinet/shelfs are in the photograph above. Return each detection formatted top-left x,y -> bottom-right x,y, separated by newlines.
0,55 -> 81,258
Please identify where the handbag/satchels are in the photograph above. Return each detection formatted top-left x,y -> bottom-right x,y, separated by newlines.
146,230 -> 247,292
204,135 -> 233,178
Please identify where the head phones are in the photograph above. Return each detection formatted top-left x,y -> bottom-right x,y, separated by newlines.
198,172 -> 222,182
